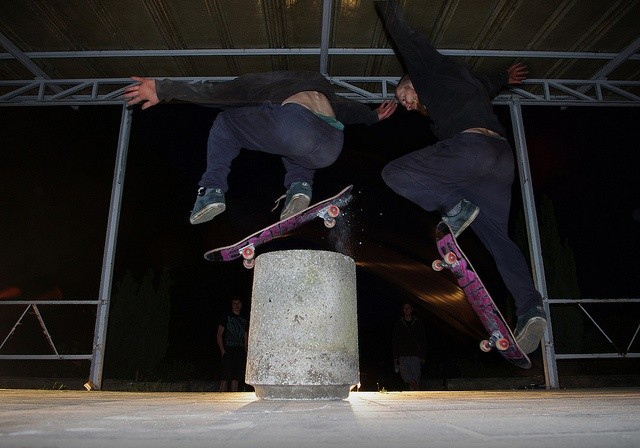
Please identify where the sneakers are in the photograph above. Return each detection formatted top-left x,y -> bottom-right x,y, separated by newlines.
440,198 -> 481,239
513,304 -> 548,355
189,186 -> 226,225
270,180 -> 313,221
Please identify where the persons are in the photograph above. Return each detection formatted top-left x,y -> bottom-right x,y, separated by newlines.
373,0 -> 548,356
392,301 -> 428,391
216,295 -> 248,392
119,69 -> 398,225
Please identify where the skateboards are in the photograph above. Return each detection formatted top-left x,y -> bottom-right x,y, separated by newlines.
205,184 -> 353,268
432,220 -> 532,369
